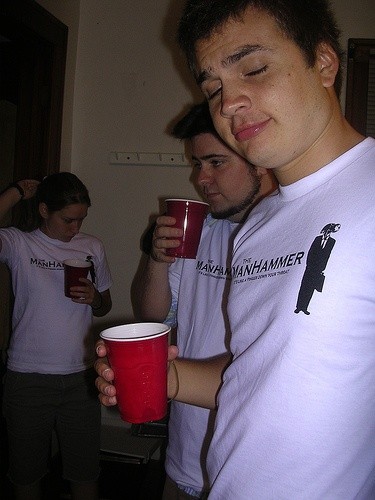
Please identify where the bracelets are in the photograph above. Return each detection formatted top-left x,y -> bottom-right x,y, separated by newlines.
167,361 -> 181,403
14,183 -> 26,201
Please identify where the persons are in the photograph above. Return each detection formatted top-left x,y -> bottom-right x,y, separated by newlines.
0,170 -> 113,500
130,104 -> 280,499
95,0 -> 375,500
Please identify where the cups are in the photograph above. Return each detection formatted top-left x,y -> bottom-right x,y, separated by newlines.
164,199 -> 210,259
63,259 -> 91,299
99,323 -> 171,424
